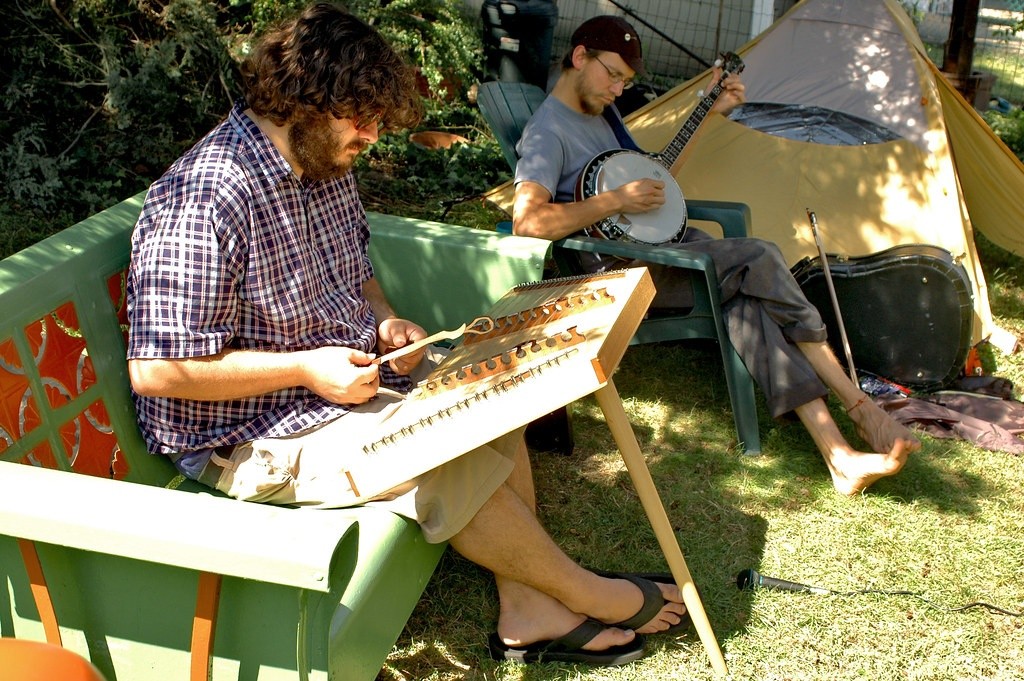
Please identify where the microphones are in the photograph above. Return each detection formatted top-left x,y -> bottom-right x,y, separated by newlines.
738,569 -> 839,597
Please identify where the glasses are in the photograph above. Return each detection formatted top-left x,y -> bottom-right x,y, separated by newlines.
355,112 -> 387,138
585,48 -> 636,90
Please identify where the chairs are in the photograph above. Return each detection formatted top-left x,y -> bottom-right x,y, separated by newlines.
477,80 -> 761,456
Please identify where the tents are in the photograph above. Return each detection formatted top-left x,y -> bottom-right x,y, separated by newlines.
471,1 -> 1024,374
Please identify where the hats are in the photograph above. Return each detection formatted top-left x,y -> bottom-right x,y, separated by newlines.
571,15 -> 648,77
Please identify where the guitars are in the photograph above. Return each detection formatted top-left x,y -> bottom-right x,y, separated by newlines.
574,51 -> 746,262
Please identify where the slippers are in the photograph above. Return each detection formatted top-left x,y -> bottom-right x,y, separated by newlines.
489,617 -> 647,667
584,567 -> 702,635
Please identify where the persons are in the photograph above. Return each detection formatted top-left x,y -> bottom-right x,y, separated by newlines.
122,6 -> 687,665
511,14 -> 923,492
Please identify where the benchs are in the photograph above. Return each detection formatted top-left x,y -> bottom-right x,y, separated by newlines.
0,188 -> 575,681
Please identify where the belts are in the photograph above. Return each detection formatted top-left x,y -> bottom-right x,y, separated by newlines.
199,443 -> 236,490
